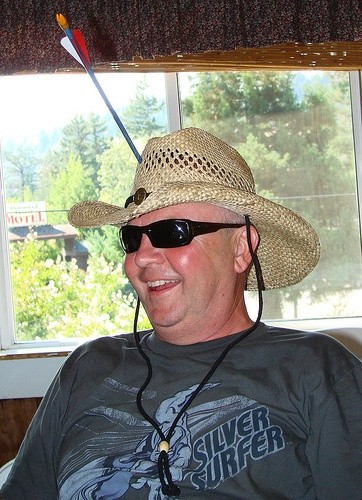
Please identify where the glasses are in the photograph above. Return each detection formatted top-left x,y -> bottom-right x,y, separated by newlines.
118,219 -> 244,255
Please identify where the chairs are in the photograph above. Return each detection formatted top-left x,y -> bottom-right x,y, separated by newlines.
0,327 -> 362,500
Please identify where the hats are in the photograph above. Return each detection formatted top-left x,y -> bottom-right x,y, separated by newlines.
67,126 -> 321,291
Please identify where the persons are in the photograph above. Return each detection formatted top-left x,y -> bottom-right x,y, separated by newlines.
0,127 -> 362,500
